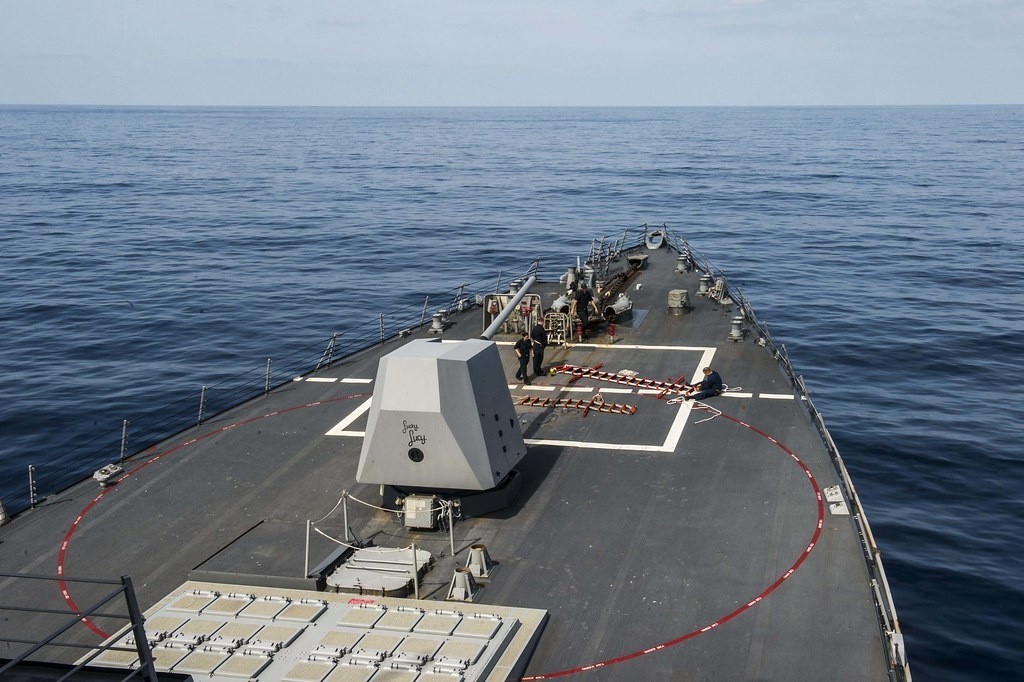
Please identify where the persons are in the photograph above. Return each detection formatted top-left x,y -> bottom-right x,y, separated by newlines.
570,284 -> 599,339
530,318 -> 548,376
514,330 -> 534,386
685,366 -> 723,402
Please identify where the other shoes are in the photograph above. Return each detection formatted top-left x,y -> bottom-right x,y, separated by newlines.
534,370 -> 547,377
685,382 -> 691,387
685,396 -> 689,401
524,380 -> 531,385
516,376 -> 522,380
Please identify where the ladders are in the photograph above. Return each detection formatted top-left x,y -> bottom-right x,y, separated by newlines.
547,363 -> 698,399
514,395 -> 636,417
